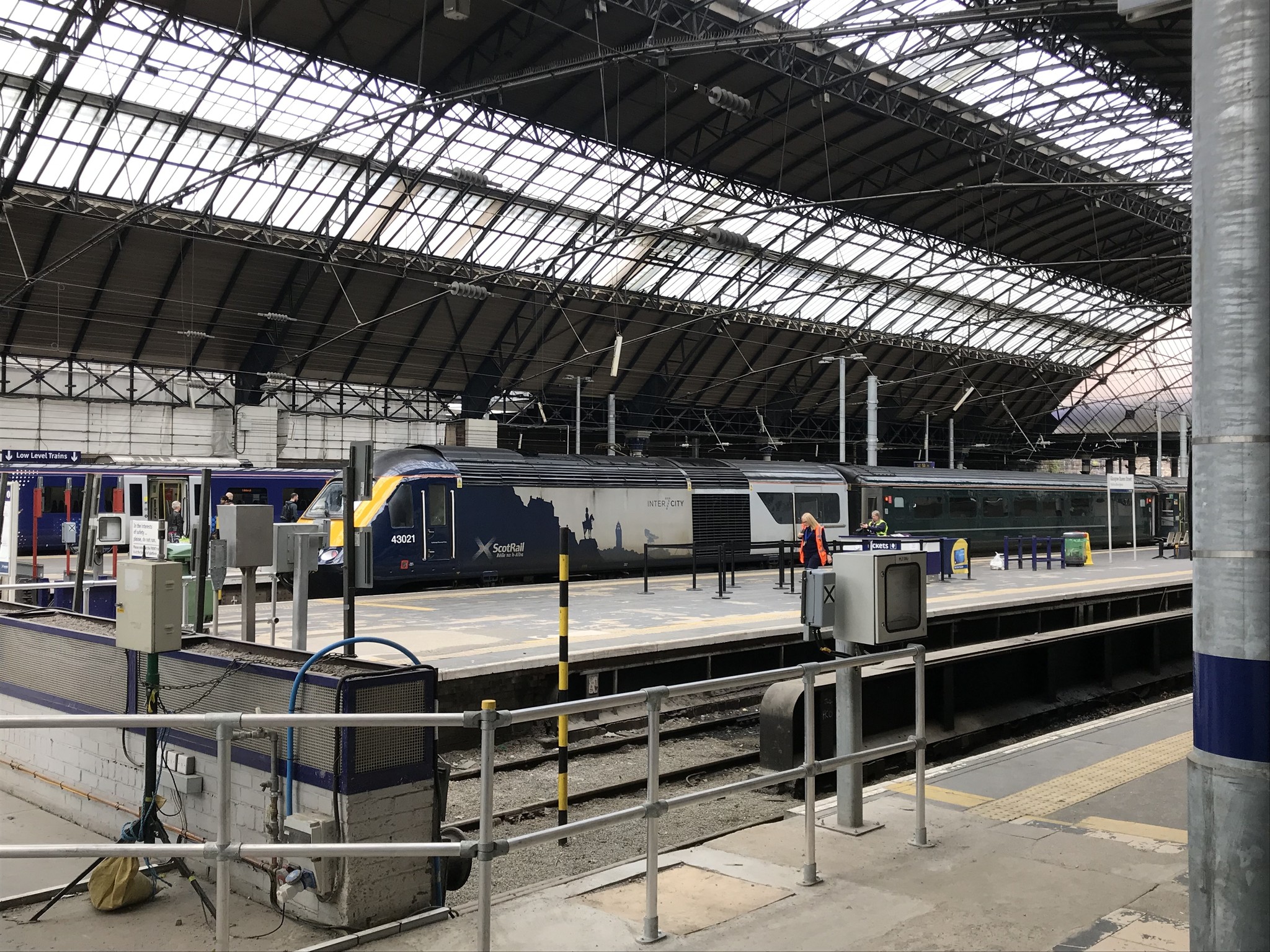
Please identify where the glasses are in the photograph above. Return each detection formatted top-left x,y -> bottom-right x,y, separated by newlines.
871,516 -> 877,518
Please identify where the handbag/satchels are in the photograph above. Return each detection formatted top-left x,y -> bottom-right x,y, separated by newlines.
990,551 -> 1005,571
168,531 -> 185,543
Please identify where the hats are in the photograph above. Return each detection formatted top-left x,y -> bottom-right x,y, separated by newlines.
226,492 -> 233,501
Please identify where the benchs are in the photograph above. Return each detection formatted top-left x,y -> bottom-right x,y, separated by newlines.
1150,531 -> 1189,560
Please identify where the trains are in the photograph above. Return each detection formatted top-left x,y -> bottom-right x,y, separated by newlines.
273,444 -> 1187,601
0,455 -> 341,592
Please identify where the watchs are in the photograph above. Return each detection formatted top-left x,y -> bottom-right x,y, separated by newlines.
827,552 -> 831,555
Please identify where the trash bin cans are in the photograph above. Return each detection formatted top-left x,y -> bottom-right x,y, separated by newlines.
1062,532 -> 1087,567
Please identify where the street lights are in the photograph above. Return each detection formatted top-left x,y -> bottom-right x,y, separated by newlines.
1144,399 -> 1180,478
819,352 -> 868,463
562,374 -> 595,455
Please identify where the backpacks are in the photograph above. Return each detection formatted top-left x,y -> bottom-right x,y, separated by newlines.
280,503 -> 295,523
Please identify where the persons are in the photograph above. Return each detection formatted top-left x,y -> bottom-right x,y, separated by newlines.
280,492 -> 300,523
860,510 -> 888,536
167,501 -> 184,544
216,491 -> 234,539
797,513 -> 833,599
